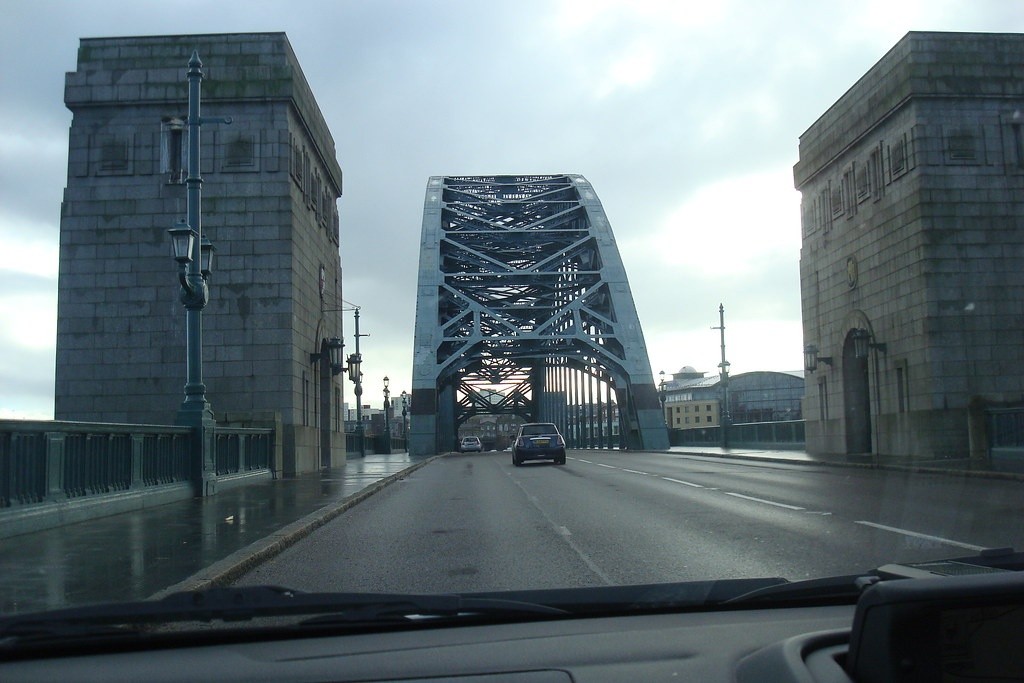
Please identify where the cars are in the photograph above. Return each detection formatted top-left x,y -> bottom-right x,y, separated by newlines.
512,422 -> 566,467
460,436 -> 483,452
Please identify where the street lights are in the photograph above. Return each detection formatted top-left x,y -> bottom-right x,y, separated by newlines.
658,369 -> 668,420
717,360 -> 733,451
382,375 -> 390,455
401,390 -> 407,453
348,353 -> 366,459
163,217 -> 217,504
